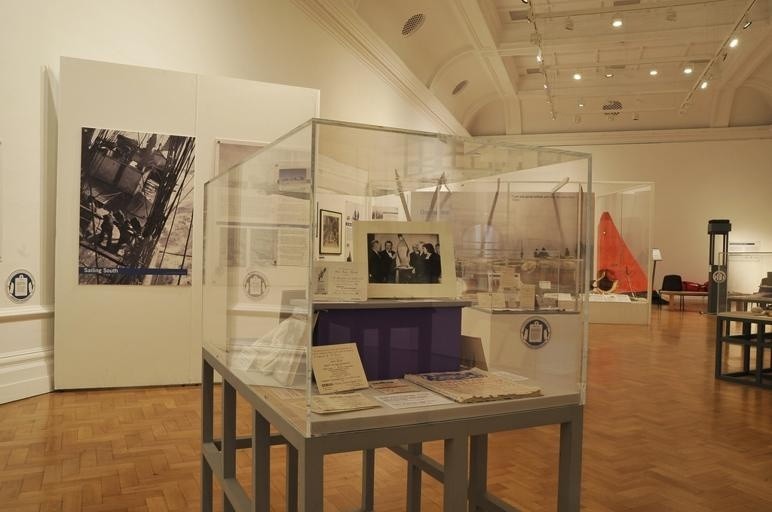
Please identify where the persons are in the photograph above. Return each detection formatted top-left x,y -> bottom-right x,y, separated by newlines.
369,239 -> 440,283
81,191 -> 142,256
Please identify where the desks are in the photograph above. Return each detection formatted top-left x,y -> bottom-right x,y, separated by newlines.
660,290 -> 772,312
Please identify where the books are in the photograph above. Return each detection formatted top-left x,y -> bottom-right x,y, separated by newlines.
405,366 -> 543,404
309,342 -> 369,394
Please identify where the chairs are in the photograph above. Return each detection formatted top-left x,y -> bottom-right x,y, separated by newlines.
657,274 -> 683,311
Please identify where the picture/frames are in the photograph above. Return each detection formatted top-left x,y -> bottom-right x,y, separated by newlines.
321,209 -> 456,300
